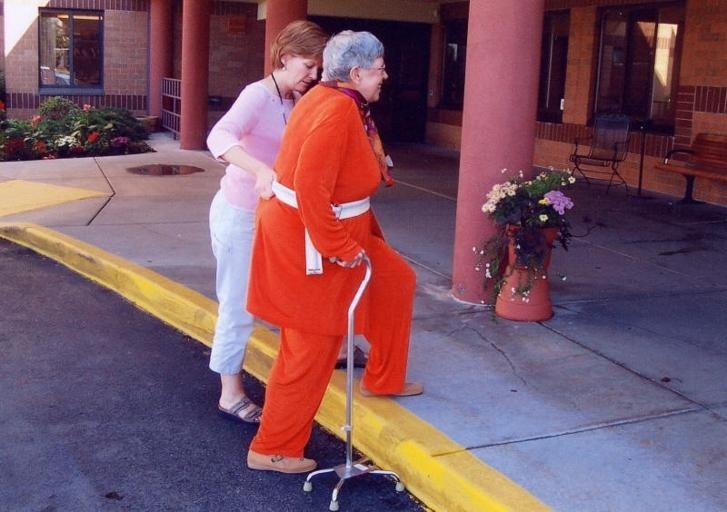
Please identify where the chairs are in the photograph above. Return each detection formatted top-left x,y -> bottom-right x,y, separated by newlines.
568,114 -> 630,196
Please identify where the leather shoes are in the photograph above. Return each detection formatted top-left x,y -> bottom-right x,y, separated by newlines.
360,377 -> 424,398
247,446 -> 317,474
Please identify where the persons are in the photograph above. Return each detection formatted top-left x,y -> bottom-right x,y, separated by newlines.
247,28 -> 422,474
205,20 -> 329,423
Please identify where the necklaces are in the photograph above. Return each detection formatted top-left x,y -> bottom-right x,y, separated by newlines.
269,69 -> 296,126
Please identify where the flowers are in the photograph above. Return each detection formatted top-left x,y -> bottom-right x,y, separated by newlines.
468,166 -> 577,313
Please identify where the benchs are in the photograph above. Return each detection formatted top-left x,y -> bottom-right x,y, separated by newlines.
655,133 -> 727,203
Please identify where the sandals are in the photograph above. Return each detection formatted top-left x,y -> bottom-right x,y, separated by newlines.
218,395 -> 263,423
335,342 -> 369,368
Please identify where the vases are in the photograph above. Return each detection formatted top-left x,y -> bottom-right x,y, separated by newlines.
495,268 -> 553,322
506,225 -> 556,271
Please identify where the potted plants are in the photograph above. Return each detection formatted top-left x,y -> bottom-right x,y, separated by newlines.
1,120 -> 29,153
